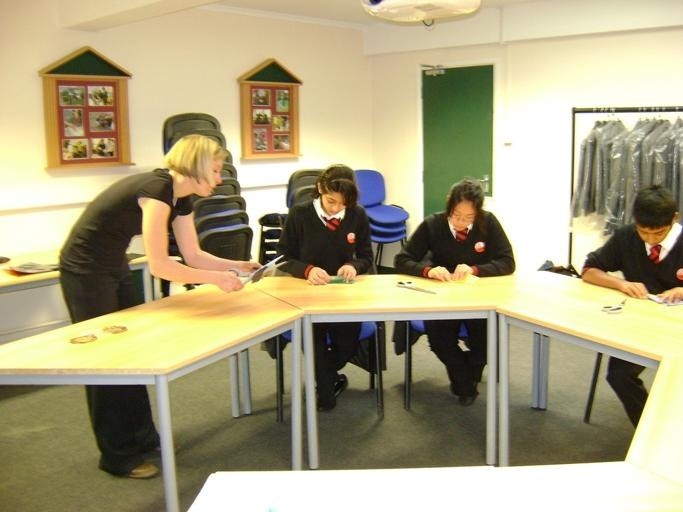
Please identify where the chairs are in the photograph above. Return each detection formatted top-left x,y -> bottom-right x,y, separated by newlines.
392,253 -> 468,411
267,167 -> 389,420
163,114 -> 252,294
356,168 -> 410,268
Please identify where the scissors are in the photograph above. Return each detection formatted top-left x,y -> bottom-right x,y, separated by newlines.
225,255 -> 287,286
397,281 -> 436,298
603,296 -> 631,313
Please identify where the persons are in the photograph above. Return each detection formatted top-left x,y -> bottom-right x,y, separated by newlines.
59,134 -> 273,479
581,184 -> 683,430
393,179 -> 515,406
277,164 -> 374,412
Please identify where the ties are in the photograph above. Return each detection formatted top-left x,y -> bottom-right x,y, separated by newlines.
325,215 -> 340,230
649,244 -> 661,264
454,229 -> 468,238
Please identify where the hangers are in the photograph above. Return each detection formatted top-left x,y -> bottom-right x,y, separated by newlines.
589,104 -> 683,122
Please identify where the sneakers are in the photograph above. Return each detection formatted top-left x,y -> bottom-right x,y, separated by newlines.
317,369 -> 349,411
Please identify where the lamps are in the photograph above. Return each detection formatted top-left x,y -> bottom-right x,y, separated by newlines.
361,0 -> 482,24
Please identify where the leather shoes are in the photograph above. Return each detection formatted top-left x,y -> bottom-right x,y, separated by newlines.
460,388 -> 479,406
127,461 -> 159,478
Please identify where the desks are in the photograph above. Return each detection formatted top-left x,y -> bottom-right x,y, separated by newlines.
497,268 -> 681,465
0,252 -> 181,351
242,269 -> 495,469
185,459 -> 680,512
2,276 -> 302,510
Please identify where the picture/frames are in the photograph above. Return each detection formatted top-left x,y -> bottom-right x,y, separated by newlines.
238,57 -> 304,163
40,48 -> 133,171
